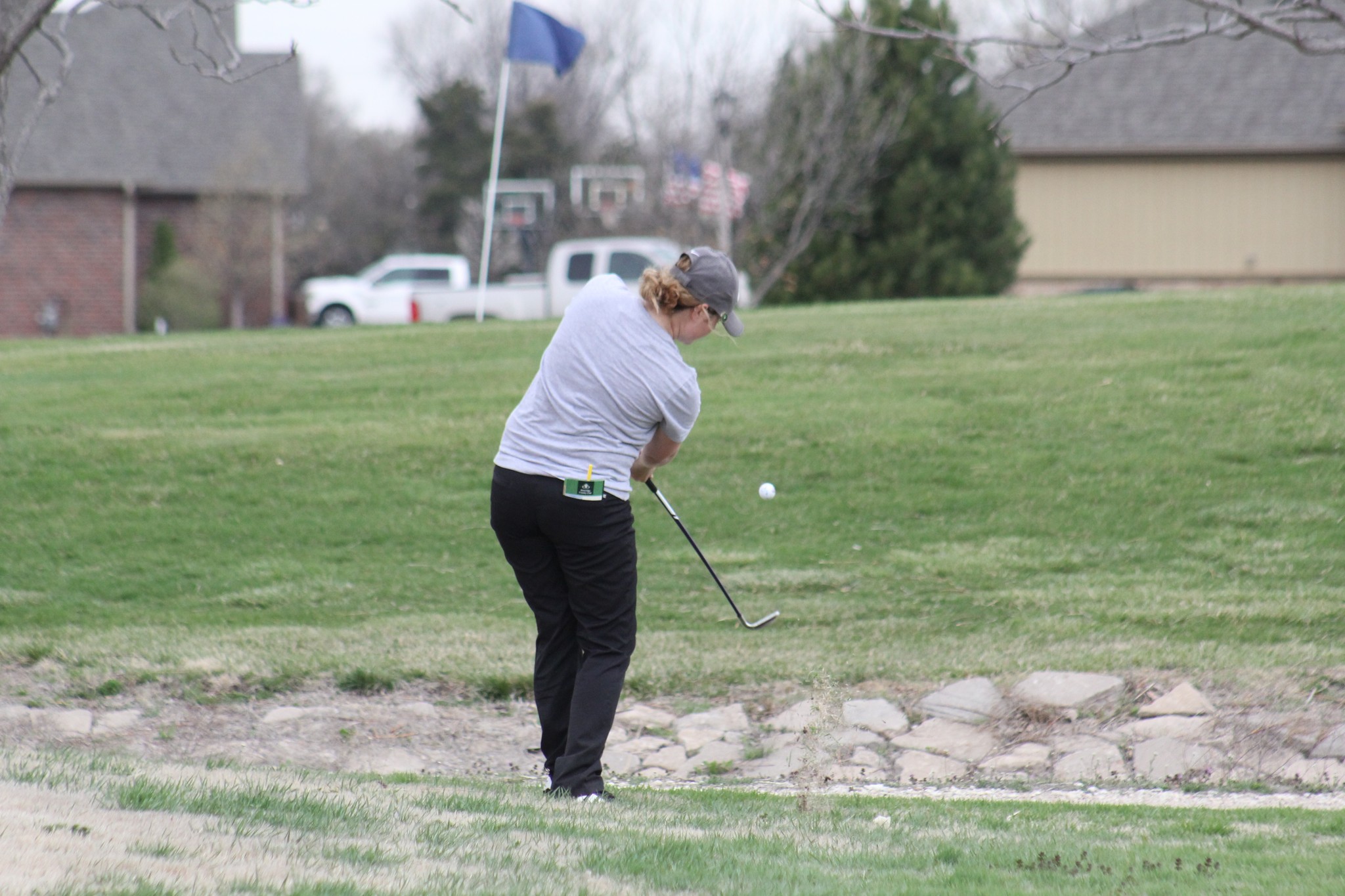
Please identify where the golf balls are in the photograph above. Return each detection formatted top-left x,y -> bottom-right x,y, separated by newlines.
758,482 -> 776,501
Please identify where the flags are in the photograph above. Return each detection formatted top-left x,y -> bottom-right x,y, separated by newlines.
661,146 -> 751,221
504,1 -> 587,79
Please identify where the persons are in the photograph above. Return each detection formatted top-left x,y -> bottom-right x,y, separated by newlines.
489,248 -> 744,803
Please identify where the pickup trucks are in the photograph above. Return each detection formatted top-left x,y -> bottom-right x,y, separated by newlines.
299,252 -> 547,328
406,235 -> 754,323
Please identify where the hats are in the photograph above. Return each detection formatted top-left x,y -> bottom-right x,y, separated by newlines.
670,246 -> 743,337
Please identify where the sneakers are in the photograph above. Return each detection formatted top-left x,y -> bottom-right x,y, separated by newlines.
575,791 -> 622,804
543,774 -> 553,793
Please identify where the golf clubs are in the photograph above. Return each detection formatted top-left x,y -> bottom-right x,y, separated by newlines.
645,478 -> 780,631
